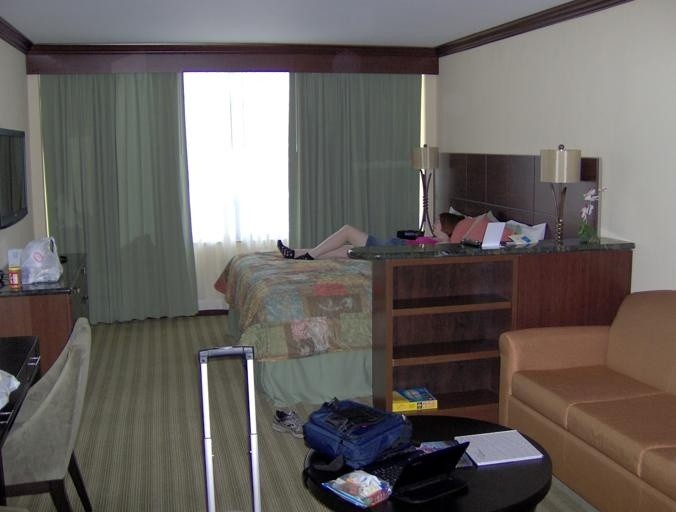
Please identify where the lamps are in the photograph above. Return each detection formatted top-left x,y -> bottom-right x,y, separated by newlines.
540,143 -> 583,242
412,143 -> 440,237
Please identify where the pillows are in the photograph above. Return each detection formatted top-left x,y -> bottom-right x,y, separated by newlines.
445,205 -> 547,250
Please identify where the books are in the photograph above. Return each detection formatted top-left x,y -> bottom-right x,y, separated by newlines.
454,428 -> 546,467
392,387 -> 438,412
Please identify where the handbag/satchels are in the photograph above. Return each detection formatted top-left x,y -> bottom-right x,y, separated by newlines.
301,396 -> 412,469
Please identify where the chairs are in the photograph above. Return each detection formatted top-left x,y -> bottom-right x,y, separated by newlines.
0,316 -> 93,511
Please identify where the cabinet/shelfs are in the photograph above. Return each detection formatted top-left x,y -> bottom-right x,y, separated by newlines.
0,253 -> 90,371
371,257 -> 517,421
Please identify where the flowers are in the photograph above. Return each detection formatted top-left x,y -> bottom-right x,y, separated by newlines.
577,183 -> 607,235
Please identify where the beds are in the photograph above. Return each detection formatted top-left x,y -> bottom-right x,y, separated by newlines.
228,152 -> 599,405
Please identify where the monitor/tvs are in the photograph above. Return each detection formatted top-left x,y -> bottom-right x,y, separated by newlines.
0,128 -> 28,230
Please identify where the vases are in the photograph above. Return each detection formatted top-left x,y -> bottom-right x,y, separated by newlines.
579,227 -> 593,242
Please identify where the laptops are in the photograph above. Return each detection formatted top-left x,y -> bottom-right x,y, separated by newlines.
364,442 -> 470,495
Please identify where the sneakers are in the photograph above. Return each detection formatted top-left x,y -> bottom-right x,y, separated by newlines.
271,407 -> 306,439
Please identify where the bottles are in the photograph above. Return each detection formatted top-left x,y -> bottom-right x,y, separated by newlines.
8,267 -> 21,292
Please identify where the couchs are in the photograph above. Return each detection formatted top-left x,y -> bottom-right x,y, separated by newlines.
496,287 -> 675,511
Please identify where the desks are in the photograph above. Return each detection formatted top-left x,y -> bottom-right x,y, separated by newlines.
0,334 -> 43,451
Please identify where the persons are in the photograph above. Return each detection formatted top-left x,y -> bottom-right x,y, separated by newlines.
277,212 -> 465,259
